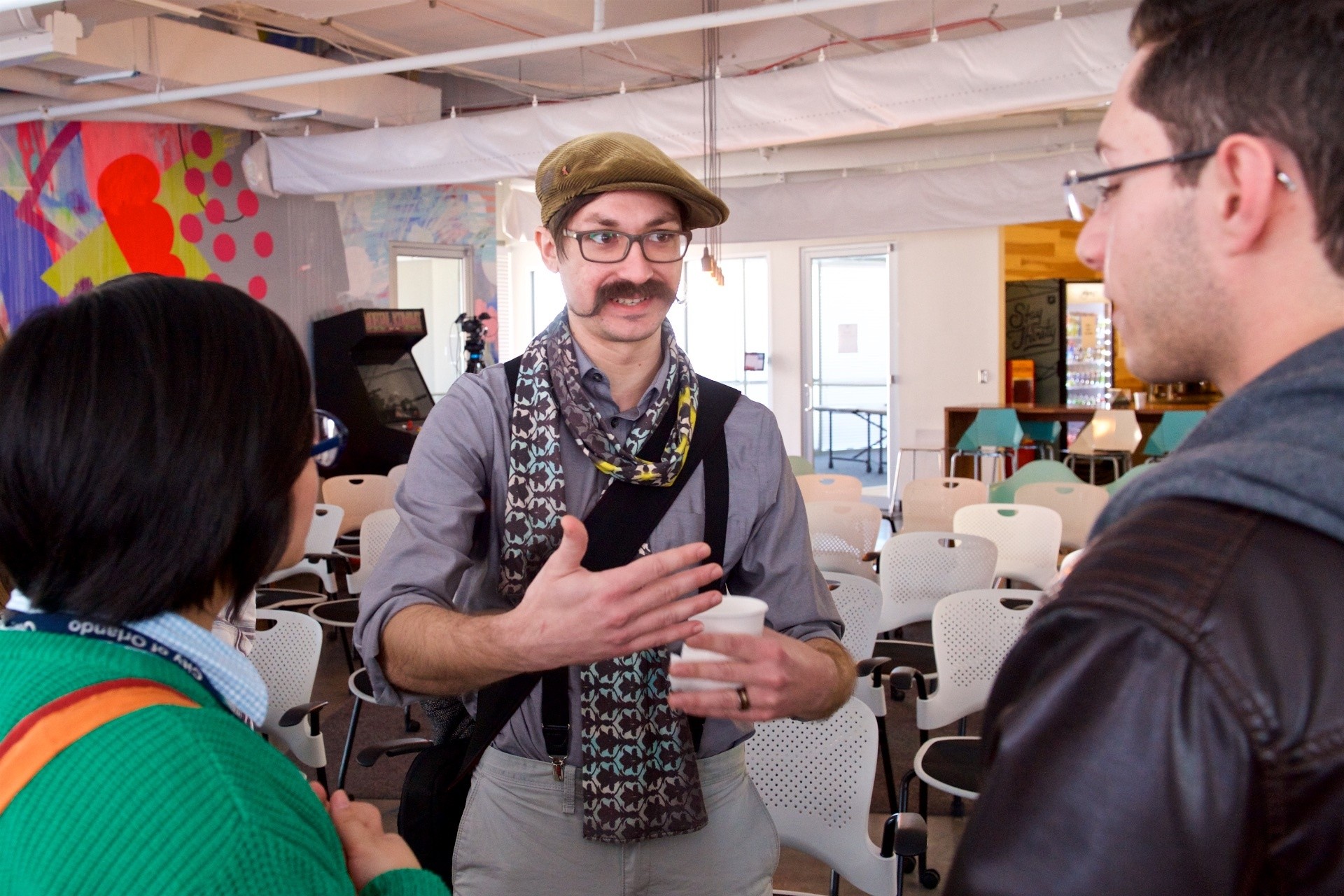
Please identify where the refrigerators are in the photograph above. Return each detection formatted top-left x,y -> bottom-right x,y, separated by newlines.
1005,278 -> 1117,459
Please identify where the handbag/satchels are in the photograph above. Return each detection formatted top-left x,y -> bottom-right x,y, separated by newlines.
395,741 -> 473,893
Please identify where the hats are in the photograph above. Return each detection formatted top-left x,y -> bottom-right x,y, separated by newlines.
535,133 -> 729,229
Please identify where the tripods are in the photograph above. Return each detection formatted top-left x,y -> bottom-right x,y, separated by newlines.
465,333 -> 486,374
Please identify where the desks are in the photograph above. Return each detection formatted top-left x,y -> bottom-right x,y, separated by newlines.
943,401 -> 1211,481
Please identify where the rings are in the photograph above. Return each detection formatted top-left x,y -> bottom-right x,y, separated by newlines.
735,686 -> 751,711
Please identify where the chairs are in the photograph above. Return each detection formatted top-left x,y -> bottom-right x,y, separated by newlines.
246,461 -> 434,799
739,461 -> 1161,896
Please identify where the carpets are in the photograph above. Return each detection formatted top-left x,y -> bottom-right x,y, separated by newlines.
262,570 -> 986,812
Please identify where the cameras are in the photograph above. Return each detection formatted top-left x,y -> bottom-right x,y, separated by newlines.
461,319 -> 483,333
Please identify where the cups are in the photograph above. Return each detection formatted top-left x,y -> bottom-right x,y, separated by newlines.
688,595 -> 769,690
1134,392 -> 1148,410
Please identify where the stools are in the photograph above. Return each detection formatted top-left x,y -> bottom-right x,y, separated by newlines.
889,427 -> 946,516
949,408 -> 1208,483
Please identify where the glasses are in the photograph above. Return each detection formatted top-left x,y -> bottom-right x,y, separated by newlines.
1063,145 -> 1297,226
561,222 -> 694,264
302,409 -> 348,468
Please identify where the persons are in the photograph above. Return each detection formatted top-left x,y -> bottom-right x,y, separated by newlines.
941,0 -> 1344,896
0,271 -> 449,896
352,132 -> 856,896
